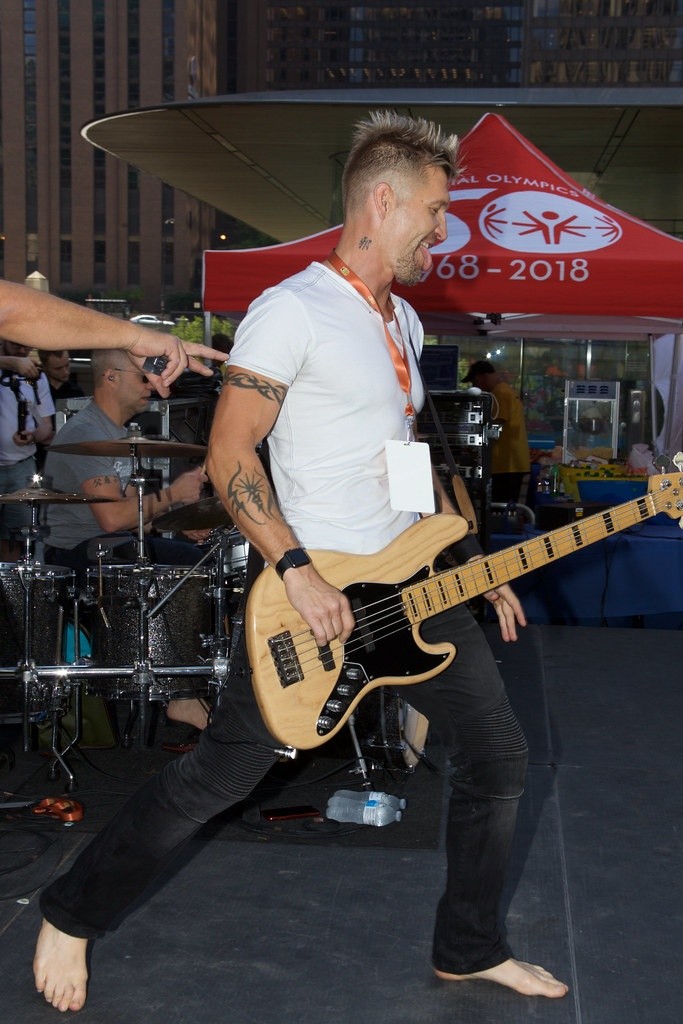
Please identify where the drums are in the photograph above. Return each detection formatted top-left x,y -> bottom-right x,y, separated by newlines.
83,558 -> 235,701
0,558 -> 80,731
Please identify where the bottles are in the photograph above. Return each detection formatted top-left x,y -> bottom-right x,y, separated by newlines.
325,797 -> 402,829
331,791 -> 408,813
532,463 -> 640,496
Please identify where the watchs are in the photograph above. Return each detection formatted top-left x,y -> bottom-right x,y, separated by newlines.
274,547 -> 311,581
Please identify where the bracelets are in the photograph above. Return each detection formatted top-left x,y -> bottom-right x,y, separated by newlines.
28,433 -> 36,446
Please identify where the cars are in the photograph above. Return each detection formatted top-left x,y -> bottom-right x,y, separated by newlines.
129,314 -> 175,326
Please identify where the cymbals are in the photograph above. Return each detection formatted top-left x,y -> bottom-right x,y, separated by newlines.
42,422 -> 211,461
0,471 -> 130,507
149,492 -> 236,533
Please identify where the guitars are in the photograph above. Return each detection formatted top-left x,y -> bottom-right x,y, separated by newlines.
244,447 -> 683,755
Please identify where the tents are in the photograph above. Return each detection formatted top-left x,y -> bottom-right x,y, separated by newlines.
197,105 -> 683,335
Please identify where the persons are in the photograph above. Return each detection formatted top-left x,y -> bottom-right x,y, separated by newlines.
461,361 -> 532,506
30,105 -> 571,1012
37,325 -> 223,734
0,339 -> 57,564
37,348 -> 85,402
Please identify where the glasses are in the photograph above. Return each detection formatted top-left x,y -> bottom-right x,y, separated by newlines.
112,367 -> 150,384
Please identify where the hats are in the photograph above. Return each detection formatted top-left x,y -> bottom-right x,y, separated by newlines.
460,360 -> 495,383
